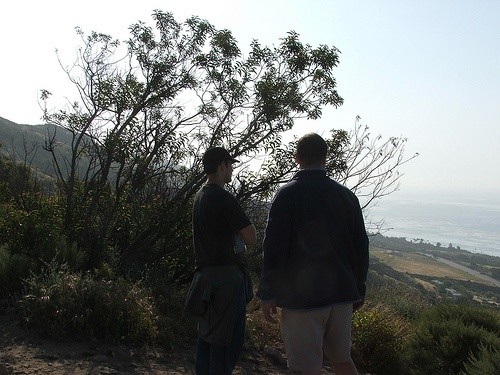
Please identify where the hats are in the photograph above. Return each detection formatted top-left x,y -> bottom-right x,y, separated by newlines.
203,147 -> 240,165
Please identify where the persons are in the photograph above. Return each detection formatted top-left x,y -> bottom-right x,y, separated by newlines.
181,147 -> 257,375
254,133 -> 370,375
